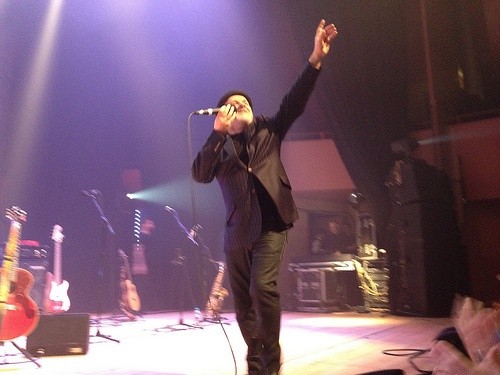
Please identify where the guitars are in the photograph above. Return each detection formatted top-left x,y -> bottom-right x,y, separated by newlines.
117,250 -> 142,311
43,224 -> 71,313
0,206 -> 40,340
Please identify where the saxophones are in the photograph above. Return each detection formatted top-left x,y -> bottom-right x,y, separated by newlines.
205,259 -> 230,316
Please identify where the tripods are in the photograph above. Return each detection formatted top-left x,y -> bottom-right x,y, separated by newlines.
90,198 -> 120,342
155,213 -> 203,331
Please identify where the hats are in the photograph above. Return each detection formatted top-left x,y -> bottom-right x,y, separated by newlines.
217,90 -> 253,115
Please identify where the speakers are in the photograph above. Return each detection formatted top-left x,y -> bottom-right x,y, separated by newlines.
26,313 -> 92,355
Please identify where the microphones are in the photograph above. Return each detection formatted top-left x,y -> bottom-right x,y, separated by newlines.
81,190 -> 96,200
165,206 -> 175,213
195,105 -> 237,115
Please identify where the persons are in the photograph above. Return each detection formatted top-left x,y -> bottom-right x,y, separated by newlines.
426,267 -> 500,375
192,18 -> 339,375
323,219 -> 353,259
406,156 -> 470,316
